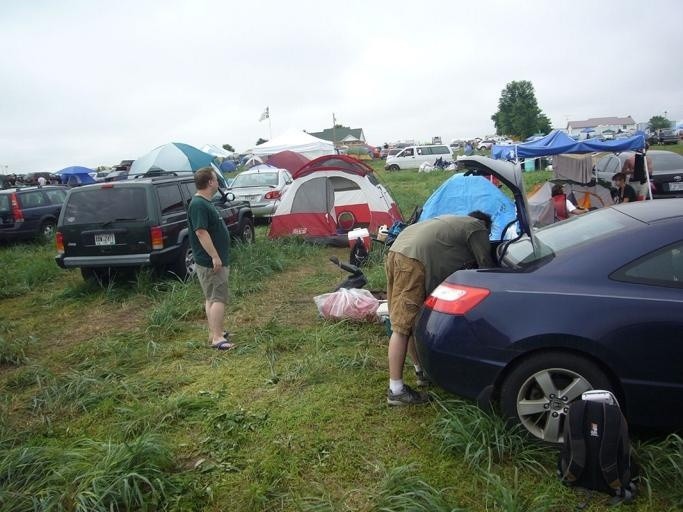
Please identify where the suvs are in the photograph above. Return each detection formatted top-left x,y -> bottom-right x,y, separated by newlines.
0,183 -> 73,245
54,169 -> 257,285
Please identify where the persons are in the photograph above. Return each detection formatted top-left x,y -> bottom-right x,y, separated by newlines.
384,209 -> 494,407
551,130 -> 665,222
186,165 -> 236,351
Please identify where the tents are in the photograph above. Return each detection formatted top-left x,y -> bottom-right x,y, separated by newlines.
267,155 -> 404,241
525,179 -> 616,231
265,150 -> 312,178
53,167 -> 98,187
244,156 -> 264,165
219,160 -> 238,171
410,173 -> 518,241
344,146 -> 372,161
126,142 -> 229,188
239,154 -> 253,164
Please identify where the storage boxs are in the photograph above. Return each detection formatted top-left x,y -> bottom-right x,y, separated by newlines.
346,227 -> 370,251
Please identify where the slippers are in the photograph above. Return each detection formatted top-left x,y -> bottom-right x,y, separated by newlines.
211,340 -> 233,351
223,332 -> 235,337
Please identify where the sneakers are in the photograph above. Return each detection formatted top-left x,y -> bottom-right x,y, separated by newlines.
387,385 -> 432,407
416,371 -> 432,386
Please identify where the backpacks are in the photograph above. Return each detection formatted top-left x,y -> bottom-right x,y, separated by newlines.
558,401 -> 639,510
348,238 -> 367,266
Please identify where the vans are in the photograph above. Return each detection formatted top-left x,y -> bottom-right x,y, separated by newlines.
384,145 -> 456,171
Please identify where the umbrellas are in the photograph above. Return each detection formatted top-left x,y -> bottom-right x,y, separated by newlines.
580,127 -> 595,133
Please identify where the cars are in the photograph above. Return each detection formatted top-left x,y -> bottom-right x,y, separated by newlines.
592,151 -> 683,200
224,168 -> 294,226
373,144 -> 421,163
7,167 -> 127,183
451,128 -> 683,150
407,154 -> 683,452
